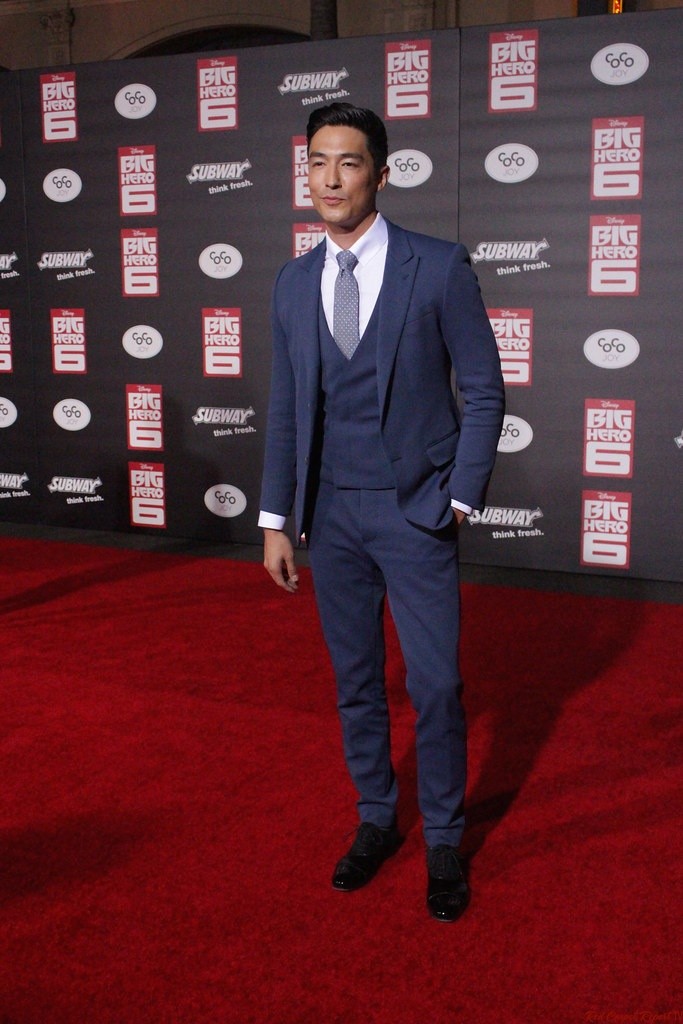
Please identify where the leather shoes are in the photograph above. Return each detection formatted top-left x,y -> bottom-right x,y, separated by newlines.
424,841 -> 470,921
330,821 -> 396,891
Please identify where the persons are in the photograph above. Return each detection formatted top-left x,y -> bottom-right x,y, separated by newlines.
259,104 -> 506,921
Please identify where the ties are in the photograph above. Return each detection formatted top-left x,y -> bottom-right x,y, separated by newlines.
334,250 -> 359,361
